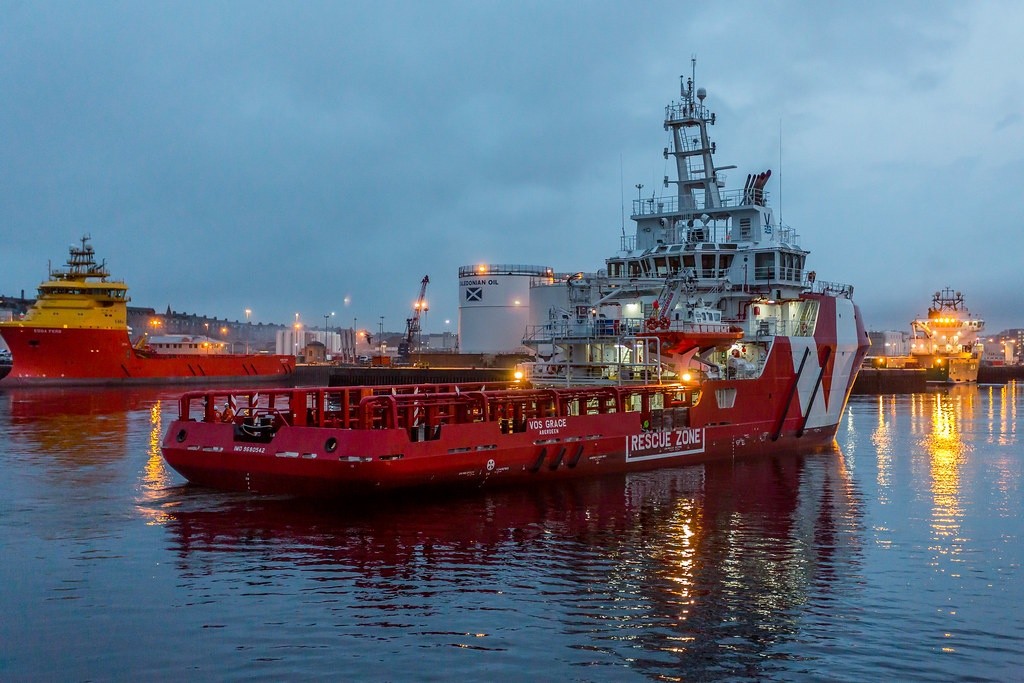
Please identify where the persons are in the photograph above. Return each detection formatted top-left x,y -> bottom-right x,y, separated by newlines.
802,324 -> 808,336
222,403 -> 233,423
535,354 -> 545,372
214,405 -> 221,422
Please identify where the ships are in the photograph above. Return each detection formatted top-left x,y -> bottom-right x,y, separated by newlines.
905,283 -> 984,384
0,232 -> 296,388
160,58 -> 870,503
